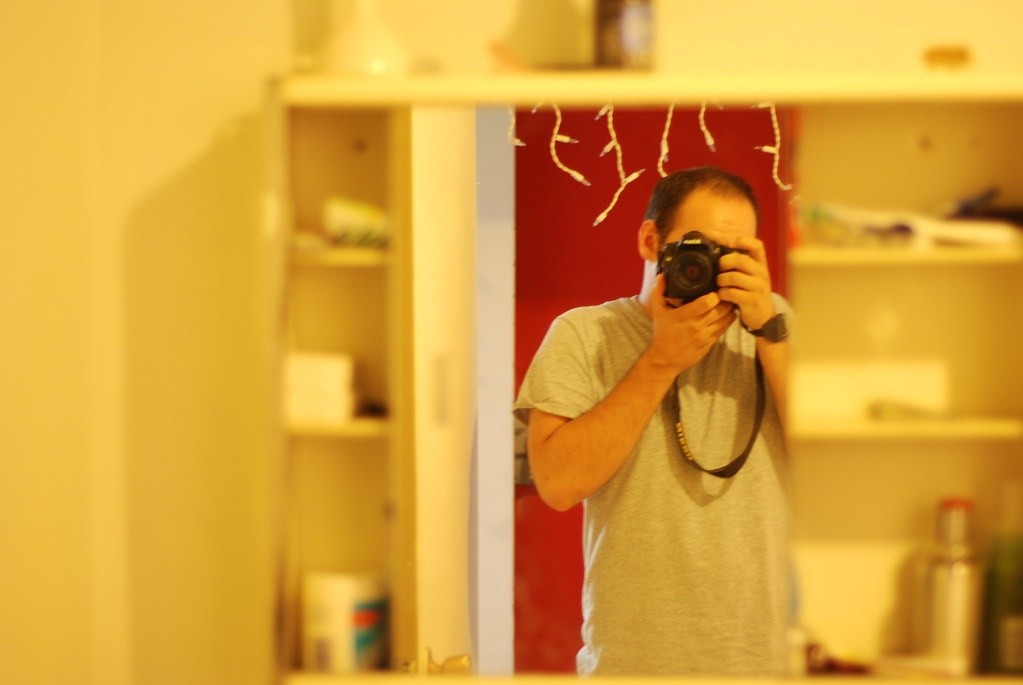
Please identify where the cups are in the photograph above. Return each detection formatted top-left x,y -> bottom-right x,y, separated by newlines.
597,0 -> 656,70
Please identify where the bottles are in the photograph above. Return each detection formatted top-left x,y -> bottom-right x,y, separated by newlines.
987,527 -> 1023,671
913,499 -> 985,673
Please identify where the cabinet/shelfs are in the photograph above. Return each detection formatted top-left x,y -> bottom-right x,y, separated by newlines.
254,68 -> 1022,685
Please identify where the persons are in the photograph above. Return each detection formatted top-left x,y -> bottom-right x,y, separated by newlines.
512,167 -> 807,684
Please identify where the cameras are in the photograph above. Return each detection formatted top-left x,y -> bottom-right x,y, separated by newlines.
652,229 -> 748,307
